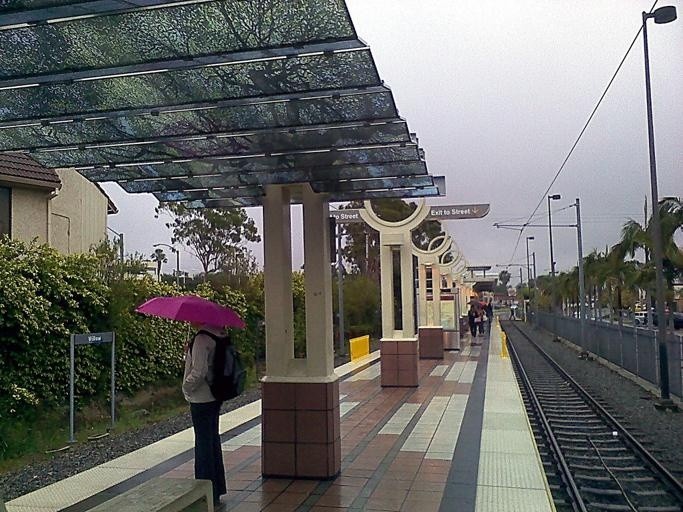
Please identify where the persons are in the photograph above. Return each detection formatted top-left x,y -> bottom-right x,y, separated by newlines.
182,322 -> 228,507
468,303 -> 493,337
509,309 -> 515,320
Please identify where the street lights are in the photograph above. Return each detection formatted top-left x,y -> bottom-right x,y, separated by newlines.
525,235 -> 537,330
640,3 -> 682,416
547,194 -> 566,344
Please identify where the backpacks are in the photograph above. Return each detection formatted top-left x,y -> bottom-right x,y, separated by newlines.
189,330 -> 246,401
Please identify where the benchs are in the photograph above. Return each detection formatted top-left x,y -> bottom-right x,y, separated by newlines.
83,474 -> 215,511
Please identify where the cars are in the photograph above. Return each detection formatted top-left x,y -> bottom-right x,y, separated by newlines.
629,303 -> 683,331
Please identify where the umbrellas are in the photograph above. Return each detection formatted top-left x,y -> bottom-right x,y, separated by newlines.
135,296 -> 245,329
467,299 -> 481,307
